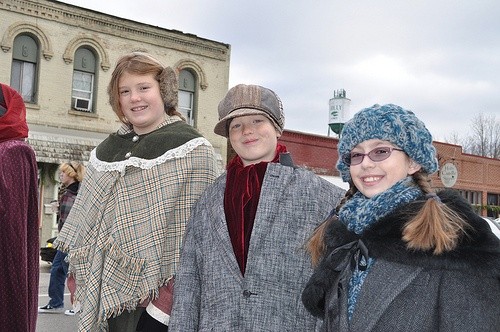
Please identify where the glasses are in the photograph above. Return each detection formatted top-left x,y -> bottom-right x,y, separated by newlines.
342,146 -> 406,166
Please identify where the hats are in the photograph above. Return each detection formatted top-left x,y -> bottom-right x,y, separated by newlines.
214,84 -> 284,135
336,104 -> 439,183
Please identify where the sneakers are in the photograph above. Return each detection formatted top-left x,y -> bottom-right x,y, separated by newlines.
37,303 -> 65,313
66,309 -> 77,315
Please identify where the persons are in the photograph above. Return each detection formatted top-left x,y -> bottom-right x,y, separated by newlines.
52,54 -> 220,332
0,82 -> 40,332
301,104 -> 500,332
167,85 -> 347,332
38,161 -> 85,316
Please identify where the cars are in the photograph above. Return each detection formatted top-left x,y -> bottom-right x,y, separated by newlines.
479,215 -> 500,241
